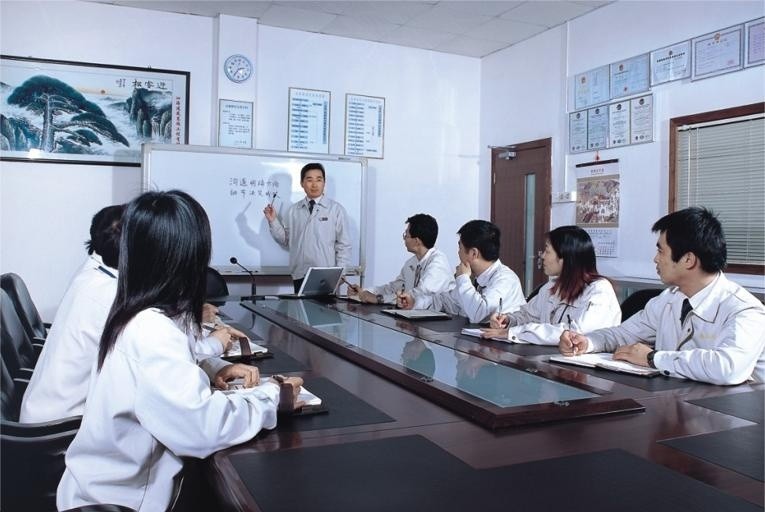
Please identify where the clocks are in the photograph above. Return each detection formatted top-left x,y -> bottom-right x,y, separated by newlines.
224,54 -> 252,83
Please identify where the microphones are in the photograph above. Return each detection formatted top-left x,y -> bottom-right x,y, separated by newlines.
230,257 -> 265,301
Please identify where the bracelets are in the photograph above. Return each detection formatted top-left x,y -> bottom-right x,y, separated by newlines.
376,295 -> 383,304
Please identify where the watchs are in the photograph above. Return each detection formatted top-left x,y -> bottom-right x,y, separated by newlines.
647,350 -> 656,369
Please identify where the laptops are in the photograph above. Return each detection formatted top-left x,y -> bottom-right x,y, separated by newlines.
278,266 -> 345,299
299,300 -> 343,328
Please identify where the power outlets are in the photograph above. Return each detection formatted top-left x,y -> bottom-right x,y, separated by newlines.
557,192 -> 565,203
565,192 -> 576,203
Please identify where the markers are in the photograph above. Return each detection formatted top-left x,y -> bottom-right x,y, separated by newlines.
271,194 -> 276,206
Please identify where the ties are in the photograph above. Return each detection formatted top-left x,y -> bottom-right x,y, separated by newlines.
414,265 -> 422,287
309,200 -> 315,214
680,299 -> 692,324
474,280 -> 479,290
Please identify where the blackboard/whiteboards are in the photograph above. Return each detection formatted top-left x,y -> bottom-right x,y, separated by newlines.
141,143 -> 369,277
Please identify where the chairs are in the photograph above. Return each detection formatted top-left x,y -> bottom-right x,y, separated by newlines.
526,283 -> 546,303
0,429 -> 79,512
621,289 -> 664,323
0,273 -> 52,344
0,352 -> 83,434
0,287 -> 44,377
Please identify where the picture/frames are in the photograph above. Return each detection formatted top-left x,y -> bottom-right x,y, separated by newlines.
0,55 -> 190,167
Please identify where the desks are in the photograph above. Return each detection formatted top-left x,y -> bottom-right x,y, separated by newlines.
613,280 -> 765,305
209,296 -> 765,511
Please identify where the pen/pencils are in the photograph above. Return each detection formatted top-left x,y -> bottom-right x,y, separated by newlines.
202,325 -> 216,331
341,278 -> 351,287
401,283 -> 405,304
498,298 -> 502,318
273,375 -> 283,382
567,314 -> 571,335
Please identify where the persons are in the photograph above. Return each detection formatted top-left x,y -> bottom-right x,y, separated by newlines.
20,202 -> 251,424
480,225 -> 622,346
264,163 -> 351,298
396,220 -> 527,325
558,204 -> 765,386
347,213 -> 456,305
55,192 -> 303,512
